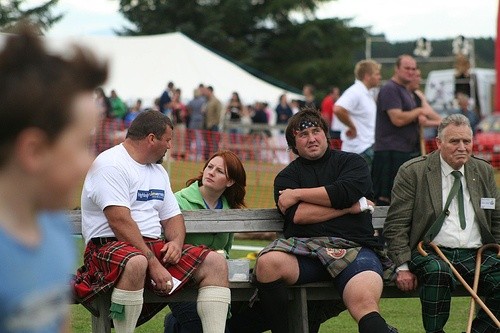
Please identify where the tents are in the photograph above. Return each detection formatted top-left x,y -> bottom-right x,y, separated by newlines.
48,31 -> 310,164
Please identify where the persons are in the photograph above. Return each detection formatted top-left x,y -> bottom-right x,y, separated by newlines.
0,25 -> 500,333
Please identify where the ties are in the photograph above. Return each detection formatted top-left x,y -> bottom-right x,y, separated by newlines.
422,171 -> 465,246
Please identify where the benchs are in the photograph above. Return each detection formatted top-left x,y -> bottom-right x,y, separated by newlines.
66,205 -> 485,333
